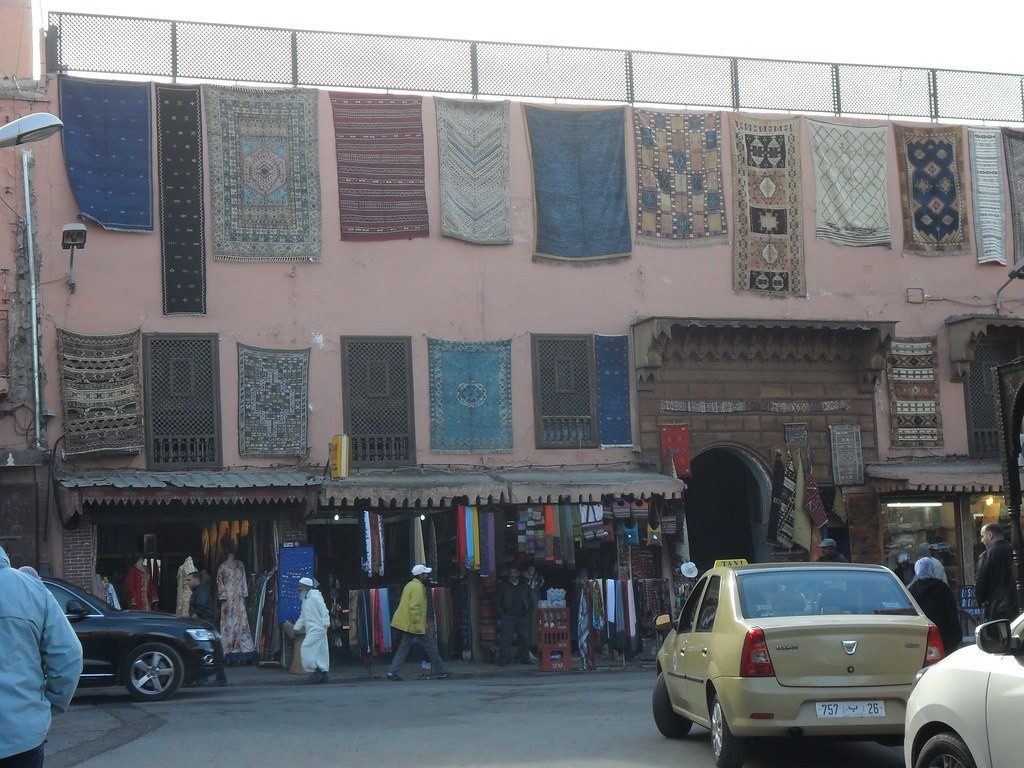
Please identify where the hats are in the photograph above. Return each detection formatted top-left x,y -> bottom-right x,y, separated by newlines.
897,553 -> 910,564
914,557 -> 934,579
299,577 -> 313,586
817,538 -> 837,547
411,564 -> 432,576
681,562 -> 698,578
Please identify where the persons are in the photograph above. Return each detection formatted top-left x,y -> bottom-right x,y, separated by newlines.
974,522 -> 1019,623
915,546 -> 949,585
293,577 -> 330,684
186,572 -> 228,688
494,566 -> 535,666
0,546 -> 84,768
215,553 -> 255,653
176,556 -> 199,616
386,565 -> 448,680
523,566 -> 545,649
127,557 -> 160,611
816,539 -> 847,562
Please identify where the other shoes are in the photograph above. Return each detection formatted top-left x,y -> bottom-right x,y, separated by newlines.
309,672 -> 322,681
521,659 -> 535,665
386,672 -> 403,681
499,658 -> 510,666
314,673 -> 329,683
436,671 -> 454,679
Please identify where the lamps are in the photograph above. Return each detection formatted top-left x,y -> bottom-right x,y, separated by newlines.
994,259 -> 1024,313
61,223 -> 87,293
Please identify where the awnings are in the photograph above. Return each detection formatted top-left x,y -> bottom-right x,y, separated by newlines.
59,470 -> 319,523
866,465 -> 1024,493
495,474 -> 685,503
309,471 -> 511,508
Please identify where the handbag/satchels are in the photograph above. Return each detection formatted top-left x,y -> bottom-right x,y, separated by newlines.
581,497 -> 676,546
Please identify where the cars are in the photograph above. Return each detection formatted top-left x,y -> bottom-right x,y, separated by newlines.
901,612 -> 1024,768
649,558 -> 945,768
39,574 -> 227,701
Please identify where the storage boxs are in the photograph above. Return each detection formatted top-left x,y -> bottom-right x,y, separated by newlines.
539,607 -> 573,672
481,604 -> 498,650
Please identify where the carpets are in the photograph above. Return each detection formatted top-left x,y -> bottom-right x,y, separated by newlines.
234,343 -> 311,458
885,337 -> 945,449
427,338 -> 513,455
594,334 -> 634,448
58,71 -> 1024,319
55,328 -> 143,458
